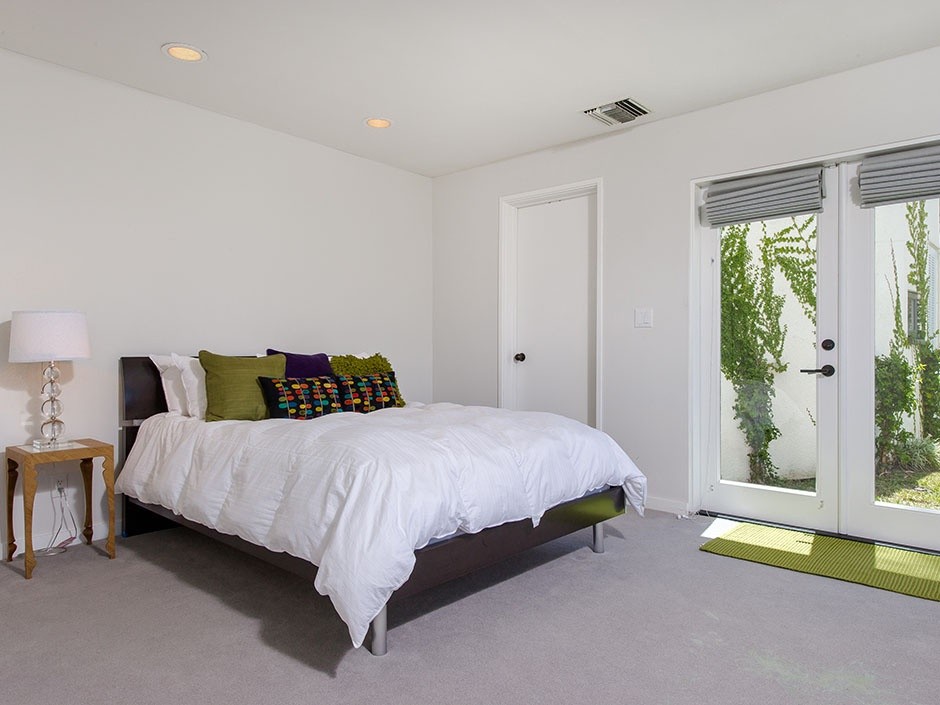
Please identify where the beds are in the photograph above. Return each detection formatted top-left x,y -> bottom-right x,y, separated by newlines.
115,353 -> 648,655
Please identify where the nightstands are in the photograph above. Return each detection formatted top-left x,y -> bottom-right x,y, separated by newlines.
6,438 -> 115,579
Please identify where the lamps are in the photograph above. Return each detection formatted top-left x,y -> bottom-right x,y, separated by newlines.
9,310 -> 94,449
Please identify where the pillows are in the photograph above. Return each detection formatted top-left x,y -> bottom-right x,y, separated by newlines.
335,371 -> 404,413
199,349 -> 286,422
149,354 -> 189,417
264,348 -> 333,378
170,351 -> 208,421
258,375 -> 344,420
328,353 -> 407,407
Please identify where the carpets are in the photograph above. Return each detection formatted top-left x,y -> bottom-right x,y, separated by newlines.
699,522 -> 940,601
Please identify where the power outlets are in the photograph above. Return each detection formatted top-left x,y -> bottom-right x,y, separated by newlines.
49,474 -> 69,498
